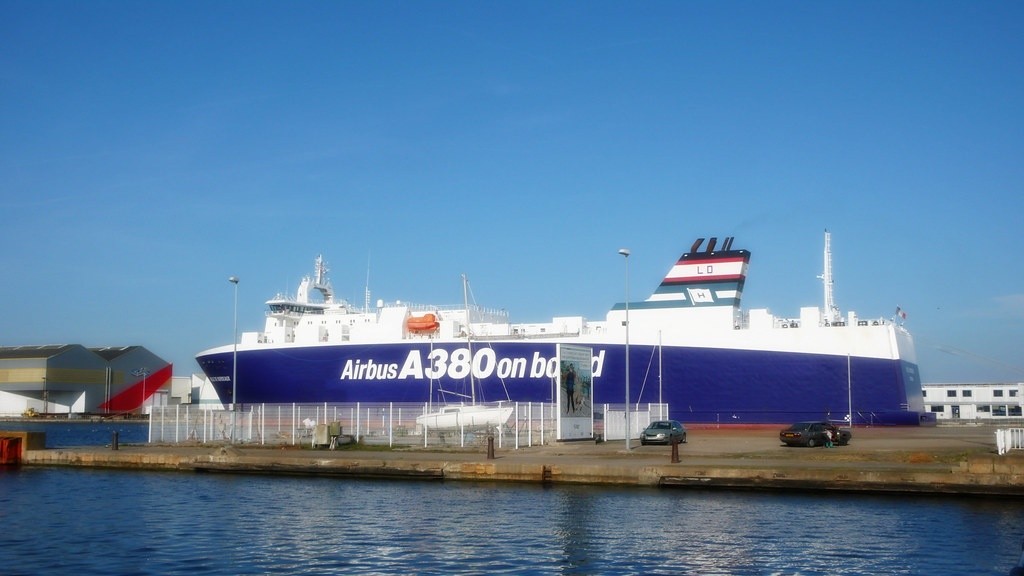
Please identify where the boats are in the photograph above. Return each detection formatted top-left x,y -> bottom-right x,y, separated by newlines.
406,313 -> 439,334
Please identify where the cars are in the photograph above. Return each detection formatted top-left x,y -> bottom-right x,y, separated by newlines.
779,420 -> 852,449
638,419 -> 687,445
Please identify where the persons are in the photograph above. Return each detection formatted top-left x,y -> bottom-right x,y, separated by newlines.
563,363 -> 576,414
822,428 -> 833,448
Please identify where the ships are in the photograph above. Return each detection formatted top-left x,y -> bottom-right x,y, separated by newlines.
191,228 -> 939,431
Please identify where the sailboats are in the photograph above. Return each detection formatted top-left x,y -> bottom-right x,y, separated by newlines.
414,274 -> 515,431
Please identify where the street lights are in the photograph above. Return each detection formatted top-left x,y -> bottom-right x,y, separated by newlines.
617,247 -> 632,452
41,377 -> 46,413
227,276 -> 240,445
